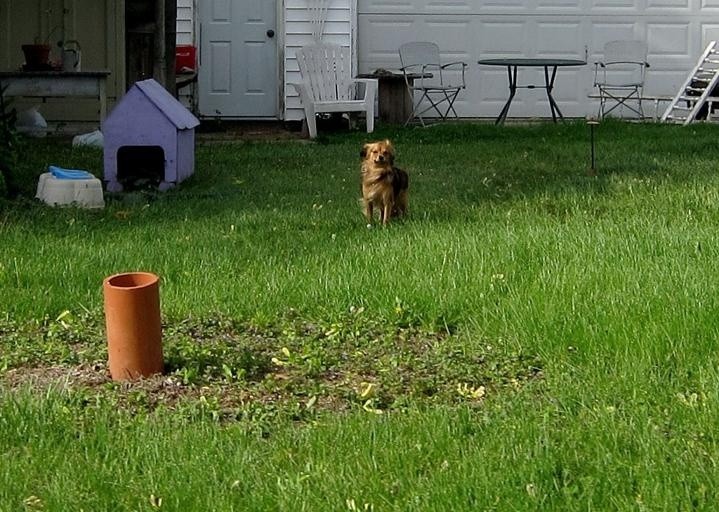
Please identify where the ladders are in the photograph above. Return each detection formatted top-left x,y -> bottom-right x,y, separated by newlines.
660,41 -> 719,126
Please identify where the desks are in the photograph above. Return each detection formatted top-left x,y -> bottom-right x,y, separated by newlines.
478,57 -> 587,129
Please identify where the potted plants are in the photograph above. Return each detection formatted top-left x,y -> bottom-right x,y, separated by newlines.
17,26 -> 58,69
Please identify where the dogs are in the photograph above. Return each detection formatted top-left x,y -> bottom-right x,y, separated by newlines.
356,138 -> 411,229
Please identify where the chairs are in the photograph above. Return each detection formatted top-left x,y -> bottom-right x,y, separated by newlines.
292,37 -> 466,141
587,39 -> 654,126
